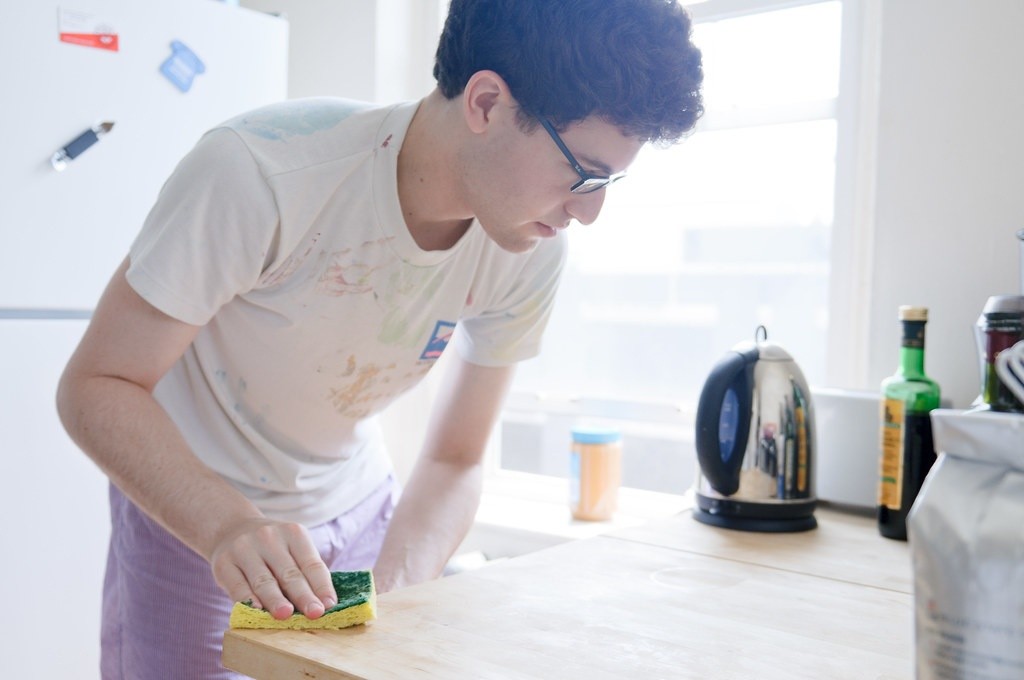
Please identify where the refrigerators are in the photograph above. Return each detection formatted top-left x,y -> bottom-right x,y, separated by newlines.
2,2 -> 290,679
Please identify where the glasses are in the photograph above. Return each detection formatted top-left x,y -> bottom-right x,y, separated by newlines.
515,88 -> 628,194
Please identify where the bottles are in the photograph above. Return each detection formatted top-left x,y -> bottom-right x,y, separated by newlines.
878,306 -> 941,542
570,429 -> 621,522
971,295 -> 1024,415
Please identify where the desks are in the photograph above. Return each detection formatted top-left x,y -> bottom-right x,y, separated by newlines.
222,492 -> 914,680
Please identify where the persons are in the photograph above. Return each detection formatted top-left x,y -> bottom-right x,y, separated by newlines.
54,0 -> 704,680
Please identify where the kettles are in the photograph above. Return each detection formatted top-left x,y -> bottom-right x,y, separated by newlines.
693,325 -> 818,532
